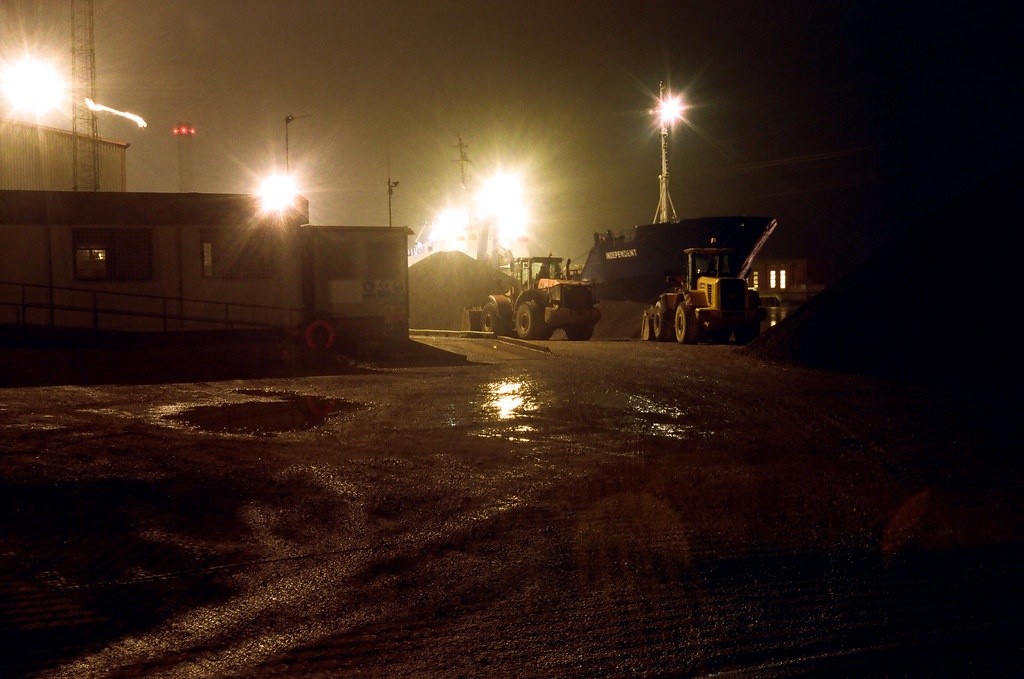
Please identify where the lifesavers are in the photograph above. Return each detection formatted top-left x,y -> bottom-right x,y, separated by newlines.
303,319 -> 336,349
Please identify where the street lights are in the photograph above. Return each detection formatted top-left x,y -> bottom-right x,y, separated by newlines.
653,101 -> 680,224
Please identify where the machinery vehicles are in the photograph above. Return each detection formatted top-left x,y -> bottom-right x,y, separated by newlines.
462,246 -> 601,341
641,237 -> 768,345
